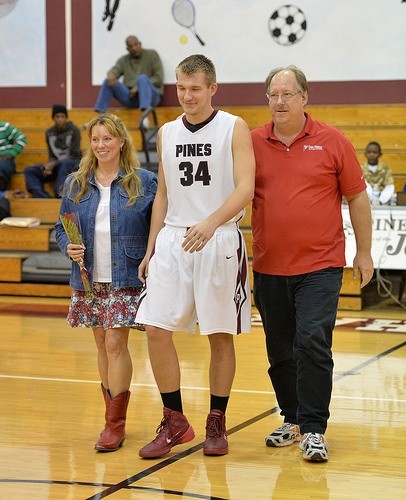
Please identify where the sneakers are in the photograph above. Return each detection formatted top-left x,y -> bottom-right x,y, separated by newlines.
203,409 -> 229,455
138,406 -> 195,457
264,422 -> 302,447
298,432 -> 330,461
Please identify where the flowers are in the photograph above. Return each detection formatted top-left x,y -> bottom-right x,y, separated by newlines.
59,211 -> 97,301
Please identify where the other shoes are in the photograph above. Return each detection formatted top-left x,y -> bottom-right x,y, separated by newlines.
83,123 -> 89,127
138,117 -> 149,130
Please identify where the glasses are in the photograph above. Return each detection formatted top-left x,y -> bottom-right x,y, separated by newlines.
271,91 -> 301,99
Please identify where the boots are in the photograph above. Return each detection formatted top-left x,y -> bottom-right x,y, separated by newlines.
94,382 -> 131,451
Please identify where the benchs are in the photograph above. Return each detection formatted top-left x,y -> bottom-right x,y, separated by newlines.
0,103 -> 406,311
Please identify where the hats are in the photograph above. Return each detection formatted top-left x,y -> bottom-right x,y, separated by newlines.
52,104 -> 67,118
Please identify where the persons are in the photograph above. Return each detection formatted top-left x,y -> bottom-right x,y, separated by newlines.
134,54 -> 256,458
0,36 -> 164,220
342,141 -> 398,206
249,65 -> 375,462
54,112 -> 158,451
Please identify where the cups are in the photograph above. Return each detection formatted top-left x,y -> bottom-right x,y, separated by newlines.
389,193 -> 398,206
